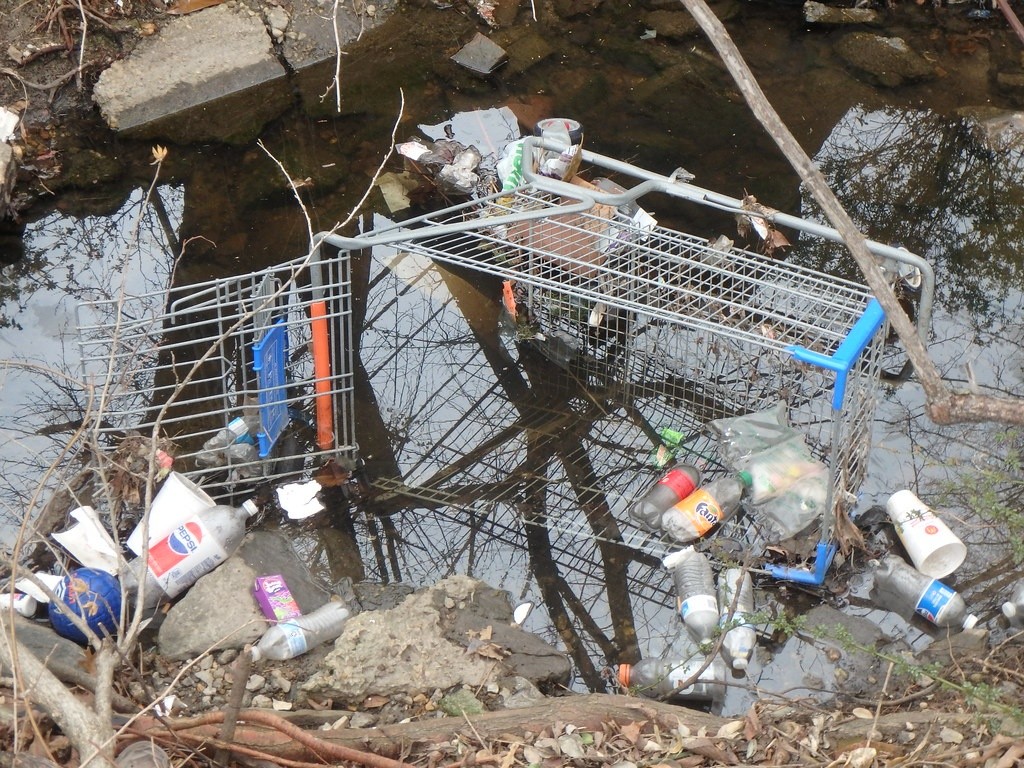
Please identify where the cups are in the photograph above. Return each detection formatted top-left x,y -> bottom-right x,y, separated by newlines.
126,472 -> 216,556
885,489 -> 966,579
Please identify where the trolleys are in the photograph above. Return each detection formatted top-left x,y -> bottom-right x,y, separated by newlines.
74,136 -> 935,586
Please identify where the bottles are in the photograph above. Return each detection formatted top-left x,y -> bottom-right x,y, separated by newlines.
250,600 -> 351,662
674,553 -> 756,669
202,416 -> 250,451
0,593 -> 36,617
1002,585 -> 1024,622
628,460 -> 752,543
620,657 -> 726,703
590,175 -> 636,204
118,499 -> 259,609
872,554 -> 977,628
